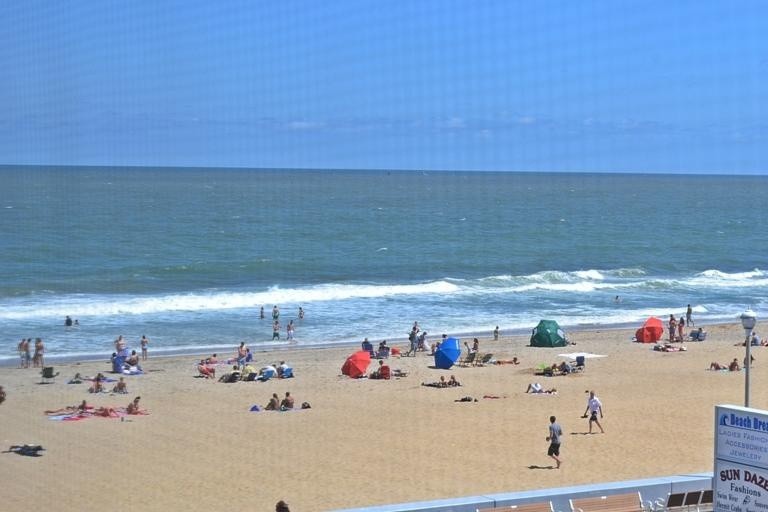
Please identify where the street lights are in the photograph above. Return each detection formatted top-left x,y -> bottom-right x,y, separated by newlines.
739,310 -> 758,408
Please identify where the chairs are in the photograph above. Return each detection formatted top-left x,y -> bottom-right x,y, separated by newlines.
228,372 -> 240,382
41,366 -> 60,383
458,352 -> 475,367
480,353 -> 494,366
198,371 -> 208,379
571,355 -> 585,372
282,368 -> 293,379
246,372 -> 256,380
259,370 -> 274,380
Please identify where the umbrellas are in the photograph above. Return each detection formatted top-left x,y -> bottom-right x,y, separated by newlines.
434,336 -> 461,369
530,319 -> 566,347
341,351 -> 371,378
636,316 -> 664,343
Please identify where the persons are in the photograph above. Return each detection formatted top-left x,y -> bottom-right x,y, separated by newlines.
432,374 -> 462,387
361,320 -> 427,379
420,375 -> 448,387
442,333 -> 447,341
614,295 -> 624,302
464,322 -> 521,365
197,305 -> 305,412
668,304 -> 768,372
275,501 -> 290,512
525,355 -> 606,469
0,315 -> 150,421
